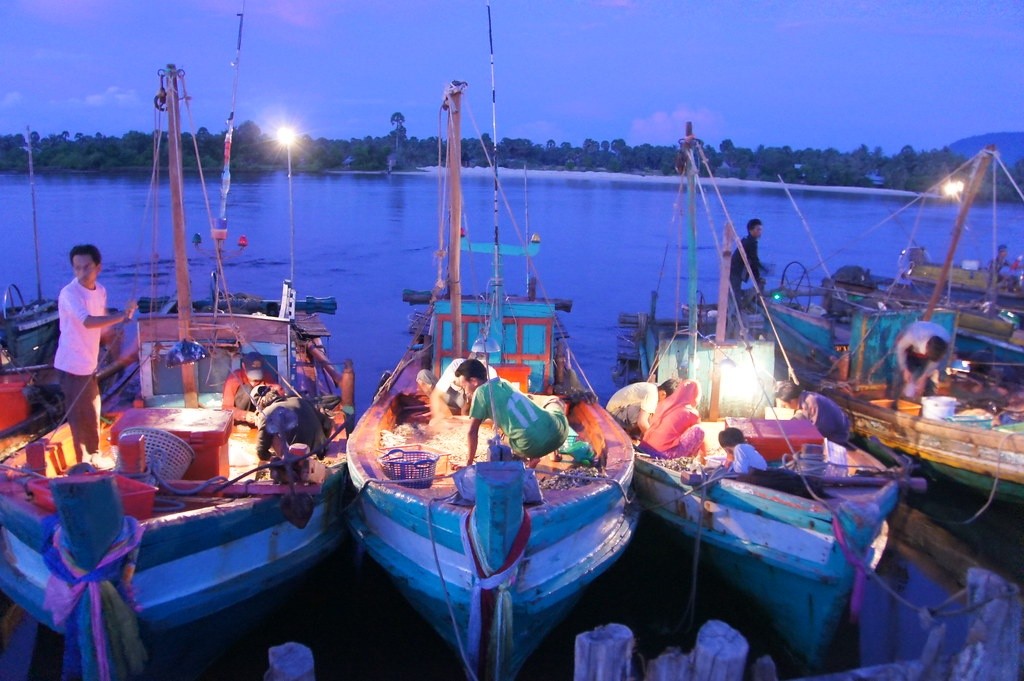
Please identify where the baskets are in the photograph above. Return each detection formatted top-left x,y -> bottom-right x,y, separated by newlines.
118,428 -> 194,481
379,449 -> 439,488
559,426 -> 579,453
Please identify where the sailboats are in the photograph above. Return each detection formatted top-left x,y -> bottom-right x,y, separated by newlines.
760,141 -> 1024,550
347,11 -> 635,681
614,119 -> 917,680
1,13 -> 354,681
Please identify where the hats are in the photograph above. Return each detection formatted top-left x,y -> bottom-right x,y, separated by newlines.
242,352 -> 265,379
250,384 -> 268,407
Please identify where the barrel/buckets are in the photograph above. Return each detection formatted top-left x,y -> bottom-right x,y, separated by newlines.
921,396 -> 957,421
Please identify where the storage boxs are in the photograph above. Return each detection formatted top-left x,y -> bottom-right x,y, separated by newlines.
111,408 -> 234,485
725,416 -> 822,459
25,471 -> 159,525
870,399 -> 921,415
947,415 -> 993,431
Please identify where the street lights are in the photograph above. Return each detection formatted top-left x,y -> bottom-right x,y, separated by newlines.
273,121 -> 301,284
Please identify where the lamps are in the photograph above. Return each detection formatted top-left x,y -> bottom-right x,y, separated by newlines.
471,338 -> 502,355
165,336 -> 209,367
950,360 -> 1024,377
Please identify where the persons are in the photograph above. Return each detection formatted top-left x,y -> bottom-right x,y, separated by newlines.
893,320 -> 949,396
719,428 -> 768,473
732,219 -> 768,314
454,358 -> 569,473
774,382 -> 849,444
222,352 -> 283,428
988,245 -> 1011,273
640,379 -> 706,465
249,385 -> 329,463
54,245 -> 139,469
605,378 -> 679,437
416,353 -> 499,419
